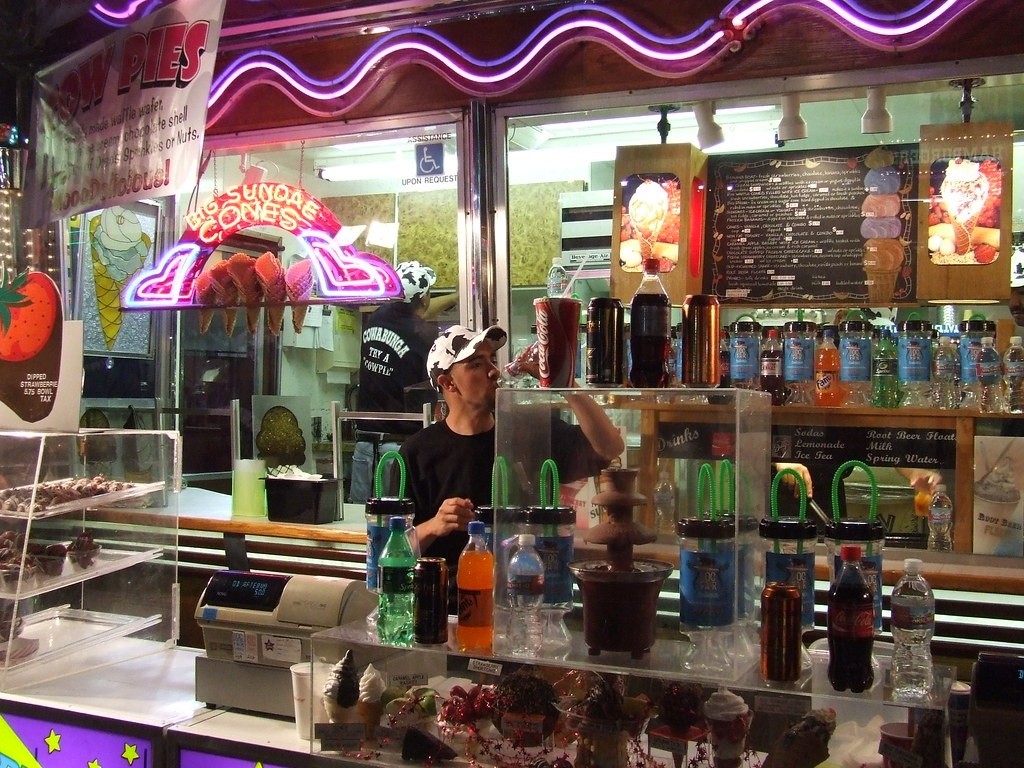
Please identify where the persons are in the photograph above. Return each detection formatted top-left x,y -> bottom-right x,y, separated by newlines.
348,262 -> 460,503
385,325 -> 626,614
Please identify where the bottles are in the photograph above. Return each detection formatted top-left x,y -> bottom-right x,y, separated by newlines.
376,517 -> 415,643
455,521 -> 495,650
927,484 -> 952,552
629,258 -> 671,387
890,559 -> 935,698
506,534 -> 545,654
547,257 -> 571,298
717,319 -> 1024,413
827,543 -> 875,693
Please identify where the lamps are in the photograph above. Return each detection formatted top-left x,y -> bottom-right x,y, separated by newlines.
778,92 -> 809,142
861,86 -> 892,135
691,100 -> 725,151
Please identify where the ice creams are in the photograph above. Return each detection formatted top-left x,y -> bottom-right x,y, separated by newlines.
705,685 -> 754,765
760,707 -> 837,768
940,159 -> 989,256
324,649 -> 386,734
629,180 -> 668,259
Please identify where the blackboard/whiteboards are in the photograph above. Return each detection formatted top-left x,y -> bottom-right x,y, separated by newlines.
703,141 -> 919,302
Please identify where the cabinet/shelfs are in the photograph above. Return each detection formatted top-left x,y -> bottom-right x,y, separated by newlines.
606,399 -> 1024,595
0,426 -> 183,688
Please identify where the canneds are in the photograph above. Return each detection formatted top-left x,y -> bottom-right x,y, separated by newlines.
585,297 -> 626,387
413,556 -> 451,646
761,581 -> 803,689
681,295 -> 721,388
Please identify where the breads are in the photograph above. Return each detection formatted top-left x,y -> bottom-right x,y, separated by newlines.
0,475 -> 132,663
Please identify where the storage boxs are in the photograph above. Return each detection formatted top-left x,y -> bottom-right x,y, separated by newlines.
258,476 -> 337,524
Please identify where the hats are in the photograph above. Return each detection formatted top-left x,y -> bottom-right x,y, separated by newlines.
426,324 -> 507,390
393,261 -> 436,304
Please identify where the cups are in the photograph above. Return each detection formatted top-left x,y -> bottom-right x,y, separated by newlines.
864,265 -> 903,303
532,297 -> 582,387
230,458 -> 267,517
674,505 -> 757,627
522,506 -> 575,610
289,660 -> 334,741
474,503 -> 521,603
365,496 -> 415,591
972,494 -> 1021,555
760,516 -> 818,629
825,519 -> 887,633
879,722 -> 918,768
